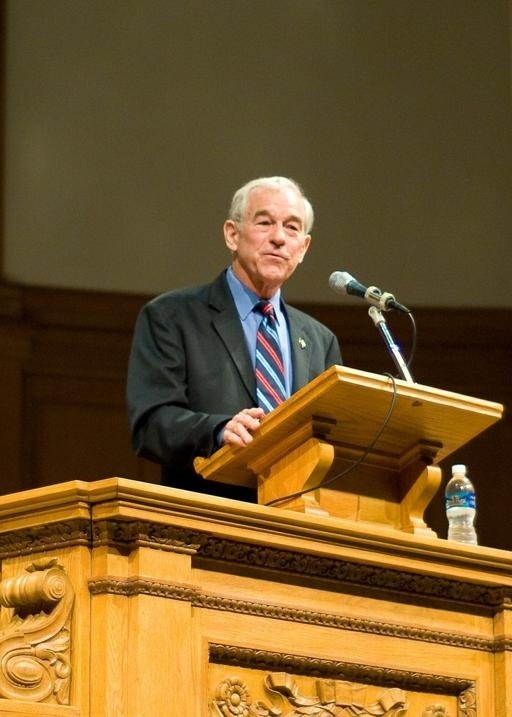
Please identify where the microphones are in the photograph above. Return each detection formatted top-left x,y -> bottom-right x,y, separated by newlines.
328,270 -> 411,314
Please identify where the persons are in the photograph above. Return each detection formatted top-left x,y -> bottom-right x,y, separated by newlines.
125,176 -> 343,504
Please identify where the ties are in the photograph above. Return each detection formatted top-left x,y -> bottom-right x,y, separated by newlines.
254,300 -> 287,417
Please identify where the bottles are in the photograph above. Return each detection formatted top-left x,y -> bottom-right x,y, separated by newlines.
445,464 -> 478,546
